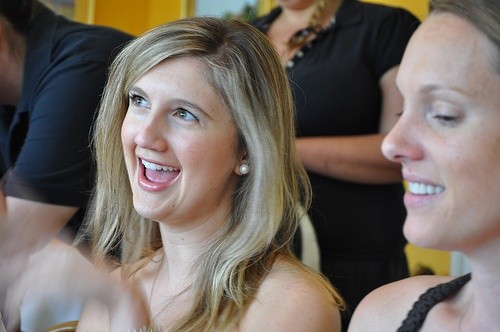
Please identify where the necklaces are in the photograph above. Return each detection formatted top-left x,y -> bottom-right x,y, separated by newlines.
286,1 -> 336,72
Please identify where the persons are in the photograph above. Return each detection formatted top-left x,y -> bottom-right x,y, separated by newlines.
347,1 -> 500,332
0,17 -> 346,332
1,1 -> 162,332
244,0 -> 423,332
